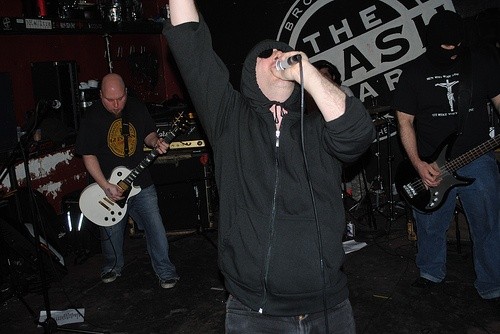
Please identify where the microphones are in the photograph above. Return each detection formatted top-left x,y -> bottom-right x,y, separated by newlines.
35,98 -> 62,109
275,54 -> 302,70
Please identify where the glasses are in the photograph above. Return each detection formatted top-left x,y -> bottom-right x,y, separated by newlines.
257,48 -> 287,58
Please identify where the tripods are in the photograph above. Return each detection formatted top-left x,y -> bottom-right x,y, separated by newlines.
0,109 -> 107,333
173,150 -> 219,251
346,97 -> 414,242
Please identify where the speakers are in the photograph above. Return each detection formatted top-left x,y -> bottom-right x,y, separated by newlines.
129,154 -> 207,235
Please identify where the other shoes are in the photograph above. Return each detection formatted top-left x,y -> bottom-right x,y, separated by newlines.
161,276 -> 181,288
101,270 -> 122,283
408,276 -> 448,290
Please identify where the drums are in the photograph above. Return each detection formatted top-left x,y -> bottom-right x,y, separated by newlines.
372,113 -> 397,143
372,150 -> 393,183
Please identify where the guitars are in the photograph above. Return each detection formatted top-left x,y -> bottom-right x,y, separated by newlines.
79,110 -> 196,227
395,133 -> 500,215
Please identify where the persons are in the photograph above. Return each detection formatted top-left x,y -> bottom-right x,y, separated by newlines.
313,64 -> 360,213
394,10 -> 500,304
161,0 -> 376,334
76,74 -> 179,289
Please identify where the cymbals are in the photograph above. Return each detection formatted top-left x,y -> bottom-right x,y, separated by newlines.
368,106 -> 392,114
